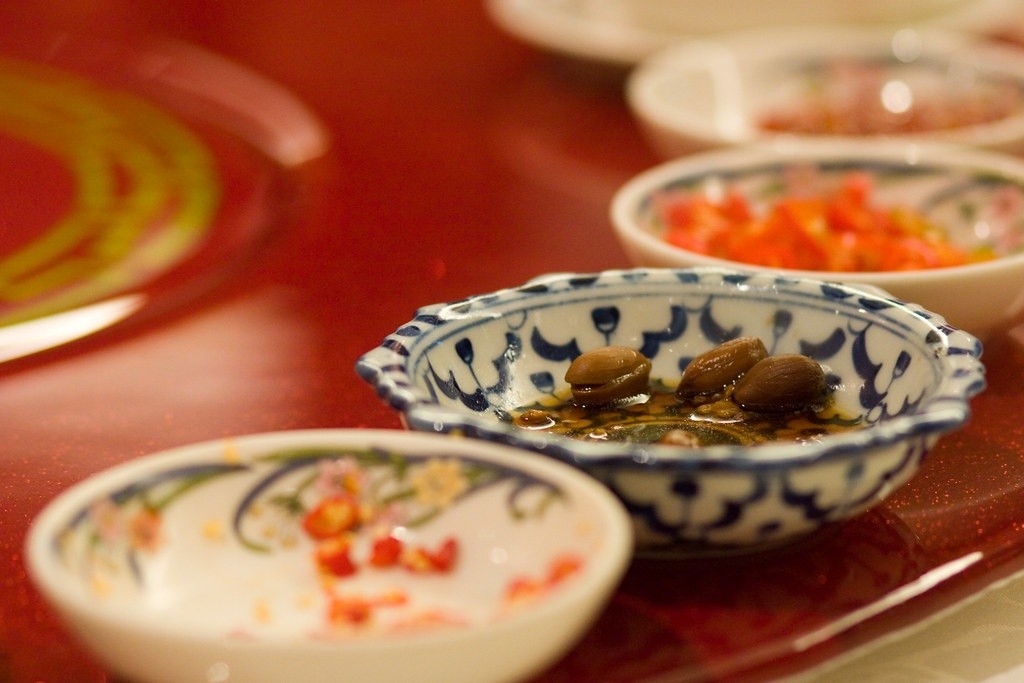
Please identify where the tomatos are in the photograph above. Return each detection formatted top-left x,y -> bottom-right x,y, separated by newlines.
657,166 -> 1004,271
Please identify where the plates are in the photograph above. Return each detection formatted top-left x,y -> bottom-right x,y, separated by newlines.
607,144 -> 1024,352
19,427 -> 637,683
622,26 -> 1024,176
354,265 -> 986,559
